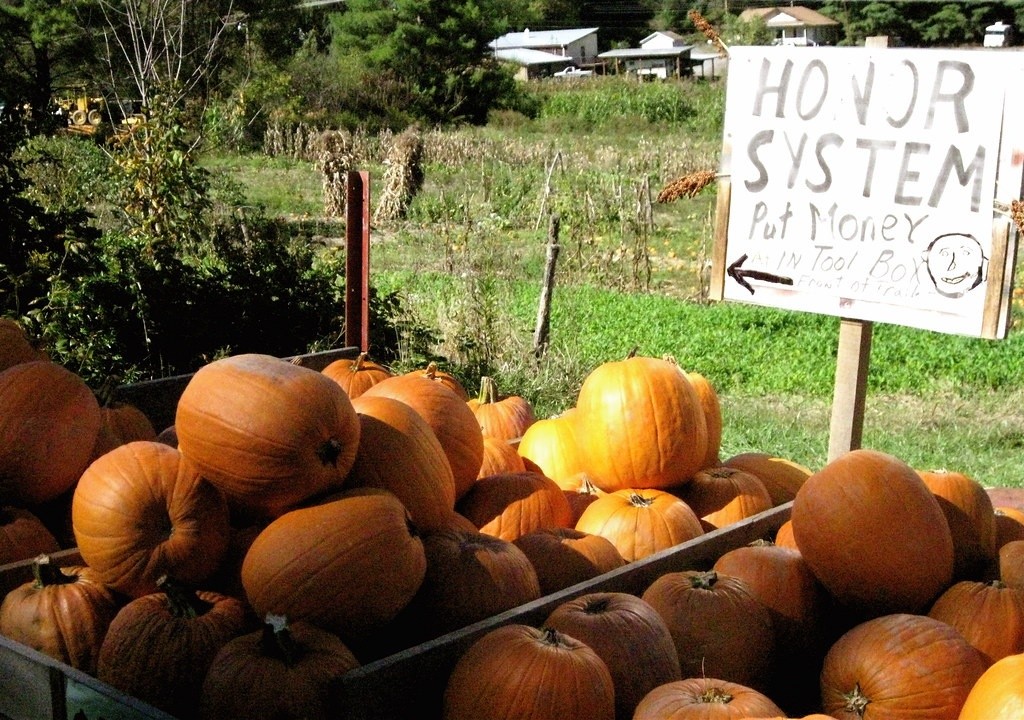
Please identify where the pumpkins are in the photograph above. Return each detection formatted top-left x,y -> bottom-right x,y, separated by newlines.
0,317 -> 814,720
442,447 -> 1024,720
